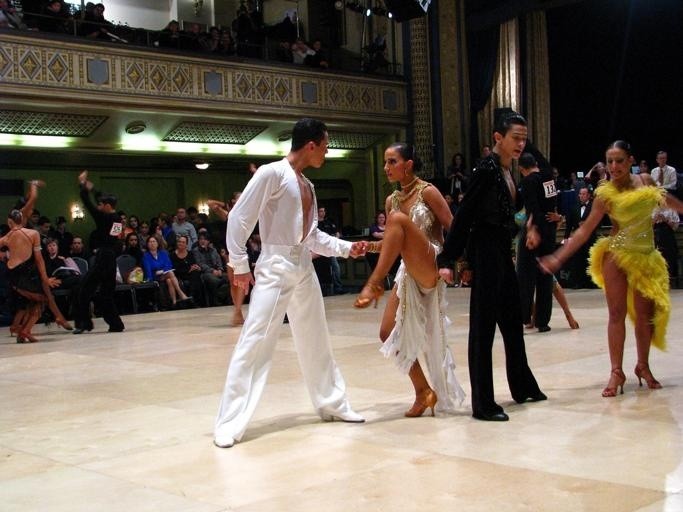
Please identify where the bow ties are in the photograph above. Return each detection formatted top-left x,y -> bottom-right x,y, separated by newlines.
580,203 -> 587,207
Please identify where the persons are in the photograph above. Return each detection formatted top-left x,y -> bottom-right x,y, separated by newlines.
207,118 -> 367,447
436,114 -> 548,423
1,146 -> 682,333
539,141 -> 682,397
354,142 -> 470,416
0,0 -> 327,67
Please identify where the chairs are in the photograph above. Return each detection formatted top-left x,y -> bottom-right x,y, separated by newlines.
50,256 -> 230,312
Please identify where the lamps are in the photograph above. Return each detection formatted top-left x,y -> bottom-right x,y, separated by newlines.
192,158 -> 213,171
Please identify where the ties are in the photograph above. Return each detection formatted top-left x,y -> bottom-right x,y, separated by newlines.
658,169 -> 665,185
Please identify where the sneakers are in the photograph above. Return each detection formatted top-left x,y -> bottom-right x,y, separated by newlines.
73,327 -> 92,334
570,320 -> 579,329
214,435 -> 234,448
539,325 -> 552,331
486,411 -> 511,421
336,289 -> 349,295
515,393 -> 548,404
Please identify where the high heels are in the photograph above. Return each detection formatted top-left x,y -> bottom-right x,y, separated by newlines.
56,318 -> 74,330
351,279 -> 383,310
322,407 -> 365,424
602,366 -> 626,398
634,360 -> 665,390
405,389 -> 438,417
9,327 -> 37,343
